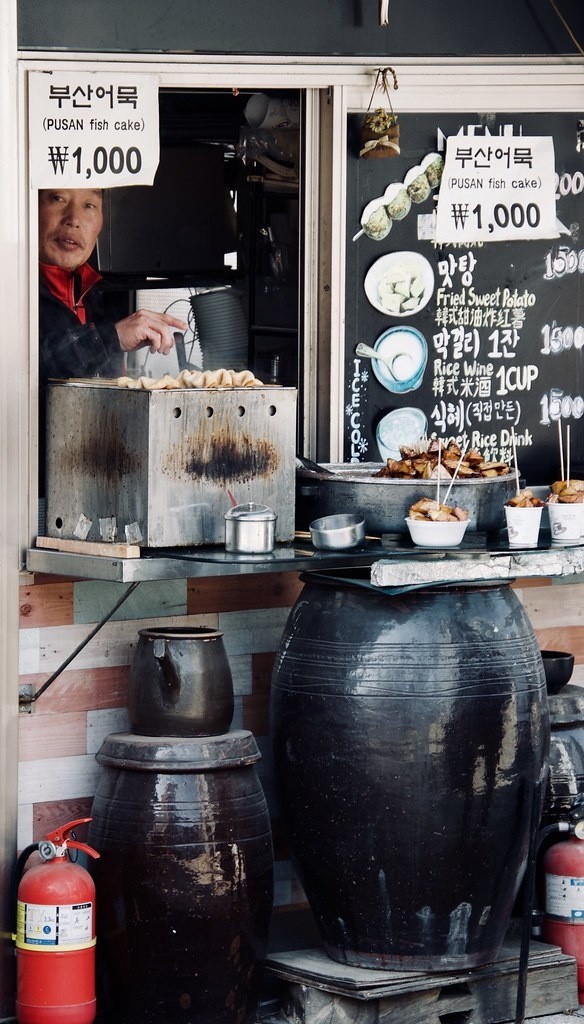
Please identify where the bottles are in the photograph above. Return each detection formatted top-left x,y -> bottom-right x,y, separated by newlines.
521,721 -> 583,940
84,769 -> 274,1024
130,625 -> 234,739
263,572 -> 553,974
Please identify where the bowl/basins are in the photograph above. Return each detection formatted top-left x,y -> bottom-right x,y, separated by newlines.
310,514 -> 366,549
541,651 -> 574,694
405,518 -> 469,547
224,504 -> 277,553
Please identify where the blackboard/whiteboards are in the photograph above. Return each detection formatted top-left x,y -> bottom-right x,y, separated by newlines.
330,81 -> 584,531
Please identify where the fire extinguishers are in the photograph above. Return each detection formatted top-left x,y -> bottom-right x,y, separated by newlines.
515,792 -> 584,1024
9,818 -> 100,1024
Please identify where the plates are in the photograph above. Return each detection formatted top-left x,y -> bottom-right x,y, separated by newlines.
187,287 -> 249,370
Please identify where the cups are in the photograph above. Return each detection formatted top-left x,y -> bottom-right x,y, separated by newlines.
548,504 -> 583,546
504,507 -> 544,547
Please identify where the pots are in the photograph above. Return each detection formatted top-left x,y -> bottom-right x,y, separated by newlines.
296,463 -> 520,533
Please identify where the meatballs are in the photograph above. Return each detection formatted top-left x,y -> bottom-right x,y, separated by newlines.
361,152 -> 443,241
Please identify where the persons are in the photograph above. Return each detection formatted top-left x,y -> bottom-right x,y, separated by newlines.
40,189 -> 188,497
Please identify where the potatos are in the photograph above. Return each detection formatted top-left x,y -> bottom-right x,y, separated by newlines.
504,489 -> 546,508
546,479 -> 584,503
408,497 -> 468,522
370,441 -> 509,483
